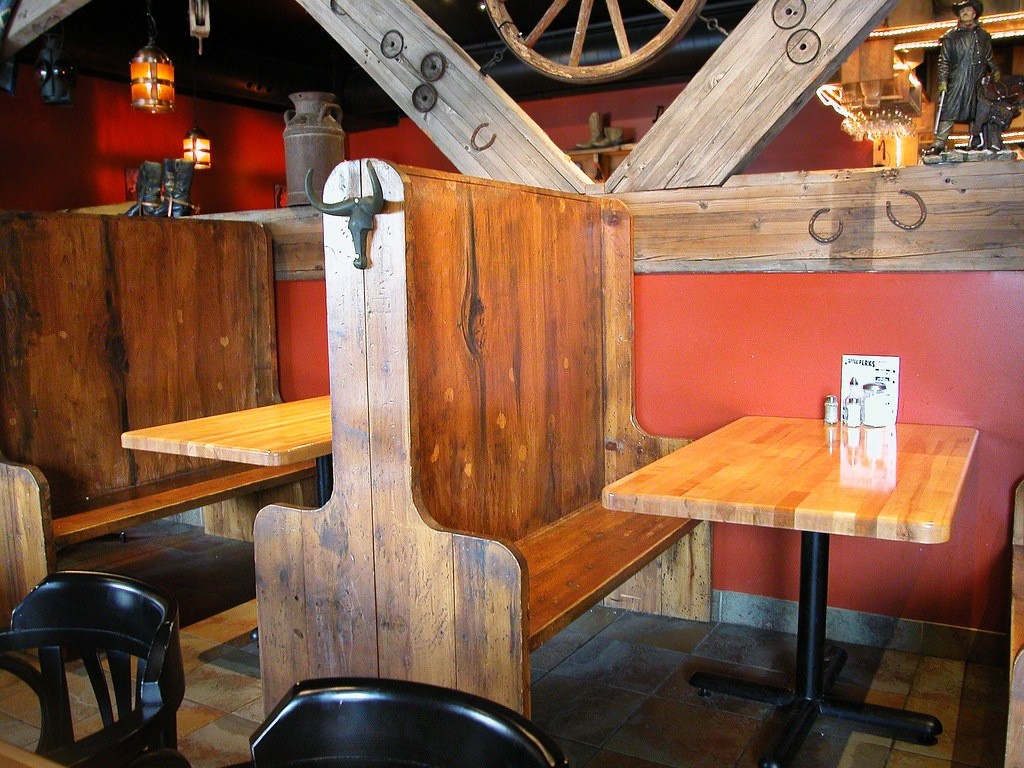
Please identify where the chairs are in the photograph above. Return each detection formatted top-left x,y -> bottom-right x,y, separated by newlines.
0,570 -> 191,768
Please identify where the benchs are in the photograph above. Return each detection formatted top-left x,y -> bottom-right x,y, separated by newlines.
254,157 -> 376,721
0,211 -> 319,660
367,155 -> 712,732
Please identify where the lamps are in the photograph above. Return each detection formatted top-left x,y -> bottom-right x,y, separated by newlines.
182,91 -> 211,171
36,14 -> 77,105
129,0 -> 174,113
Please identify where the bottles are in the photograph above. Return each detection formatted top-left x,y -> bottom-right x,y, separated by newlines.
845,378 -> 862,404
862,383 -> 887,428
846,399 -> 861,427
823,395 -> 838,424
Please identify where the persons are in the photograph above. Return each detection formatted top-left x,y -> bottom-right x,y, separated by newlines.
117,158 -> 194,217
920,0 -> 1001,155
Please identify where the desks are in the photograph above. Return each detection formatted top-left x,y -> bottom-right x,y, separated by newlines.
121,395 -> 333,512
602,416 -> 980,768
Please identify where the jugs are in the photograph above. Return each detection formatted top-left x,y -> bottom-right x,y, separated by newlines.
283,91 -> 346,207
